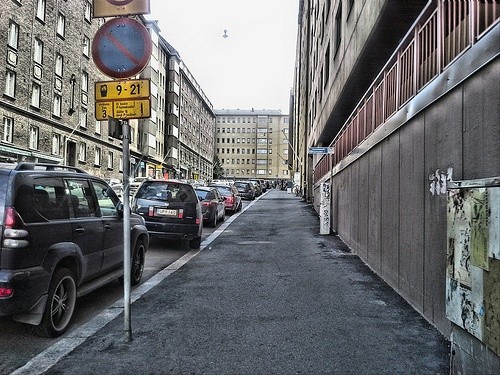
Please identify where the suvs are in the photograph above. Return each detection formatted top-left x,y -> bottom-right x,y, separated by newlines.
128,177 -> 204,249
0,159 -> 150,337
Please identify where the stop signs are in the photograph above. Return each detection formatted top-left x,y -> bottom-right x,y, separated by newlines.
90,17 -> 153,82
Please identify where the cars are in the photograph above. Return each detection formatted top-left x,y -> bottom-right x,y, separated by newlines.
192,178 -> 273,226
99,176 -> 148,202
